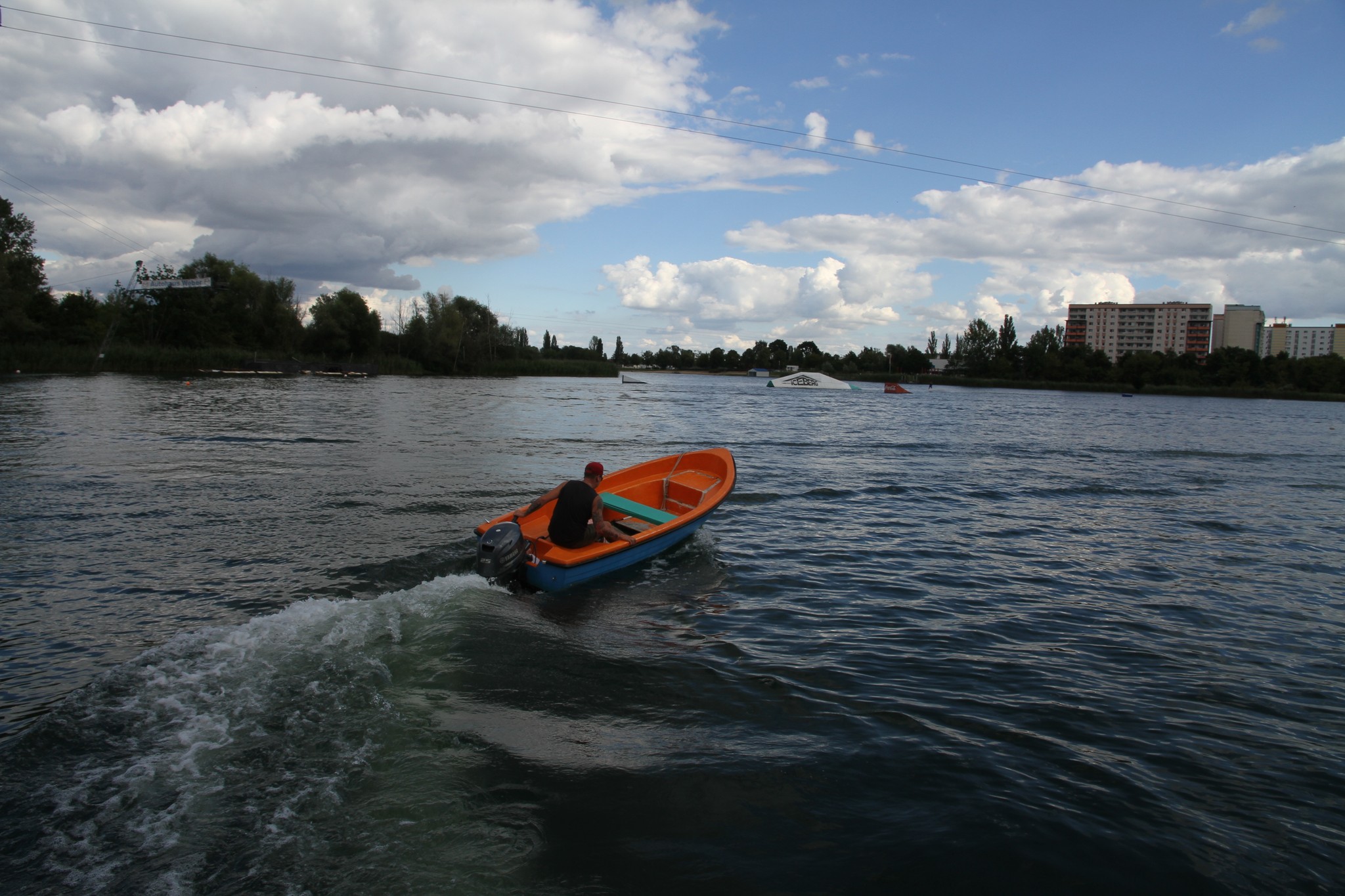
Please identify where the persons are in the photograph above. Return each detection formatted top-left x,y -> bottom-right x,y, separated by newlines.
513,461 -> 637,549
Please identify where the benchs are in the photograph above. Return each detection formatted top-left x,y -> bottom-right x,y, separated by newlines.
599,492 -> 679,526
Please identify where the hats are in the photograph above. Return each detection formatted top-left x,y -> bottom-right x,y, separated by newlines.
585,462 -> 608,476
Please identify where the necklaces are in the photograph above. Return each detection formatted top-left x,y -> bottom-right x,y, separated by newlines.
583,479 -> 593,487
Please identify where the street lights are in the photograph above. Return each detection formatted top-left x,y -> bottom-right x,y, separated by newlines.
887,352 -> 892,375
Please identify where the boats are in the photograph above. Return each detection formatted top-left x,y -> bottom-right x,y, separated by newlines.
471,445 -> 738,597
